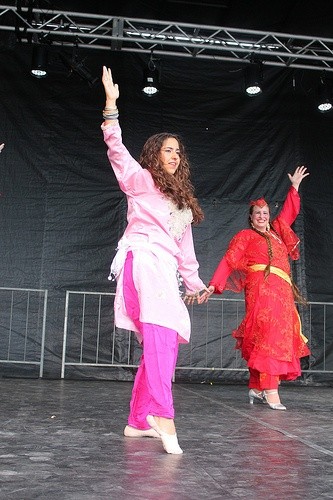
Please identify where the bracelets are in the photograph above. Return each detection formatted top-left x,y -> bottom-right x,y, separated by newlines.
103,107 -> 120,120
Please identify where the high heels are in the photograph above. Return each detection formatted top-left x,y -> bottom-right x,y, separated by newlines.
263,389 -> 286,411
249,388 -> 266,405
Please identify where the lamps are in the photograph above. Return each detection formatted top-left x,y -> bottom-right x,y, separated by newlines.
142,64 -> 159,96
60,49 -> 100,88
242,64 -> 265,97
316,73 -> 332,113
29,43 -> 48,78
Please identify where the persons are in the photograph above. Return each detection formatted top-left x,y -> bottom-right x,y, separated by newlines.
200,166 -> 312,410
102,66 -> 211,456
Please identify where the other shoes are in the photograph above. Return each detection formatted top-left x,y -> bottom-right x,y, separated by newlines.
146,415 -> 183,454
123,425 -> 160,437
